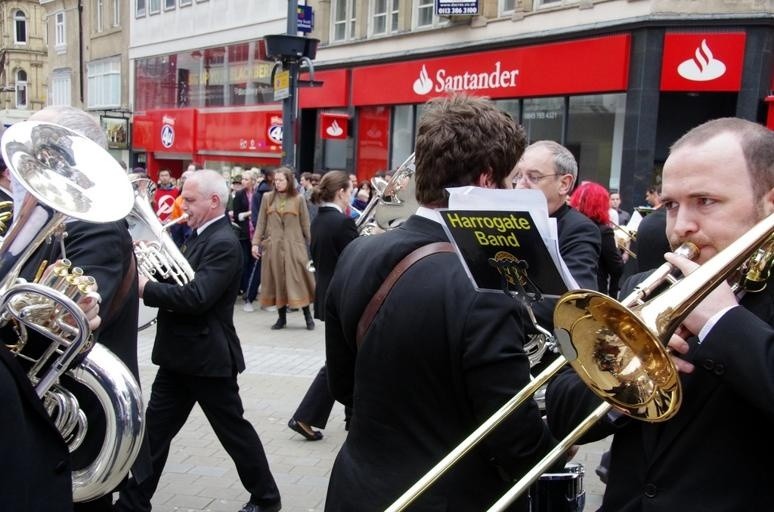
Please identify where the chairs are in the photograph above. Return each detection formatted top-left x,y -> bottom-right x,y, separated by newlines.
244,302 -> 298,312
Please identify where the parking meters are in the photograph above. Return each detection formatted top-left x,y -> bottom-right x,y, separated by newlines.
382,212 -> 774,512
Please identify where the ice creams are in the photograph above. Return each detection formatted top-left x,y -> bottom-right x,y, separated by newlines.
125,177 -> 195,312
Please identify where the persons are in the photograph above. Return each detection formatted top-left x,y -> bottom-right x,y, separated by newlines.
118,160 -> 191,226
222,166 -> 413,311
2,157 -> 13,238
2,259 -> 102,511
565,174 -> 672,301
110,169 -> 282,511
2,104 -> 140,511
288,171 -> 359,441
251,167 -> 316,330
324,94 -> 579,511
546,117 -> 773,511
514,140 -> 602,385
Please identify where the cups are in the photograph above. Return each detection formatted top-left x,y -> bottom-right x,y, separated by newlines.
512,171 -> 566,188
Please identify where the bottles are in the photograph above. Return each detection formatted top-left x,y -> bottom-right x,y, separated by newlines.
232,174 -> 242,186
133,167 -> 149,178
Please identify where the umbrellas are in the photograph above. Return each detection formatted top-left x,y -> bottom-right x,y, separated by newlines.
238,499 -> 282,512
287,416 -> 323,440
596,464 -> 608,483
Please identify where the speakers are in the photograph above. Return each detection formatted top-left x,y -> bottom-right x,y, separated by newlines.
271,305 -> 286,329
302,304 -> 315,330
